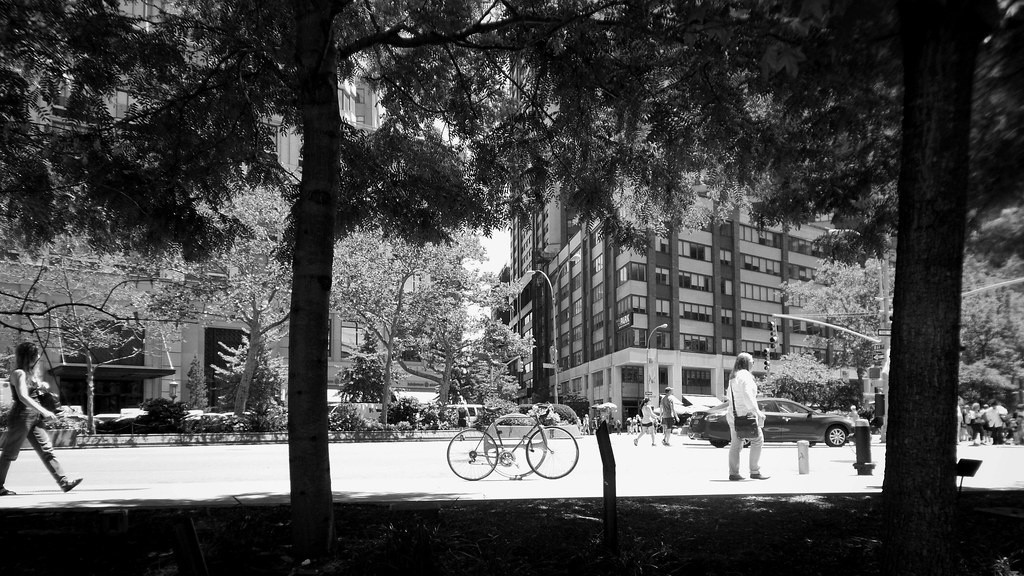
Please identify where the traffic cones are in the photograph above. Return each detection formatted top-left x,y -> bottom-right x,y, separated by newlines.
853,418 -> 875,475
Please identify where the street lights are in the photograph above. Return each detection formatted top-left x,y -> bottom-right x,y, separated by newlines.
632,322 -> 669,402
522,255 -> 582,404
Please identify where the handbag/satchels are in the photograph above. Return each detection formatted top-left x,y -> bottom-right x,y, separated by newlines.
38,386 -> 63,420
734,416 -> 759,439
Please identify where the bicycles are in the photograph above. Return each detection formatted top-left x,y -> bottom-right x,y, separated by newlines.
446,401 -> 580,481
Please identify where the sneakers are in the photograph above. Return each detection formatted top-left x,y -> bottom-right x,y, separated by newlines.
63,478 -> 83,493
0,487 -> 16,496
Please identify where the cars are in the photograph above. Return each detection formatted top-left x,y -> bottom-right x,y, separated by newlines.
689,395 -> 857,447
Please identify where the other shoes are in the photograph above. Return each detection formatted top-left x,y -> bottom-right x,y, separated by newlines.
750,474 -> 770,480
728,474 -> 746,481
634,439 -> 638,446
651,444 -> 656,446
662,439 -> 669,446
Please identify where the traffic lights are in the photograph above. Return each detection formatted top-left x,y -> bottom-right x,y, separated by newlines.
767,319 -> 778,350
764,347 -> 770,372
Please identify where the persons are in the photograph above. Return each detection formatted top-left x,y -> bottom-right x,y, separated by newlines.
37,381 -> 62,429
574,413 -> 661,436
850,403 -> 859,417
725,351 -> 770,480
0,342 -> 83,496
660,386 -> 680,446
957,395 -> 1023,445
634,396 -> 657,446
